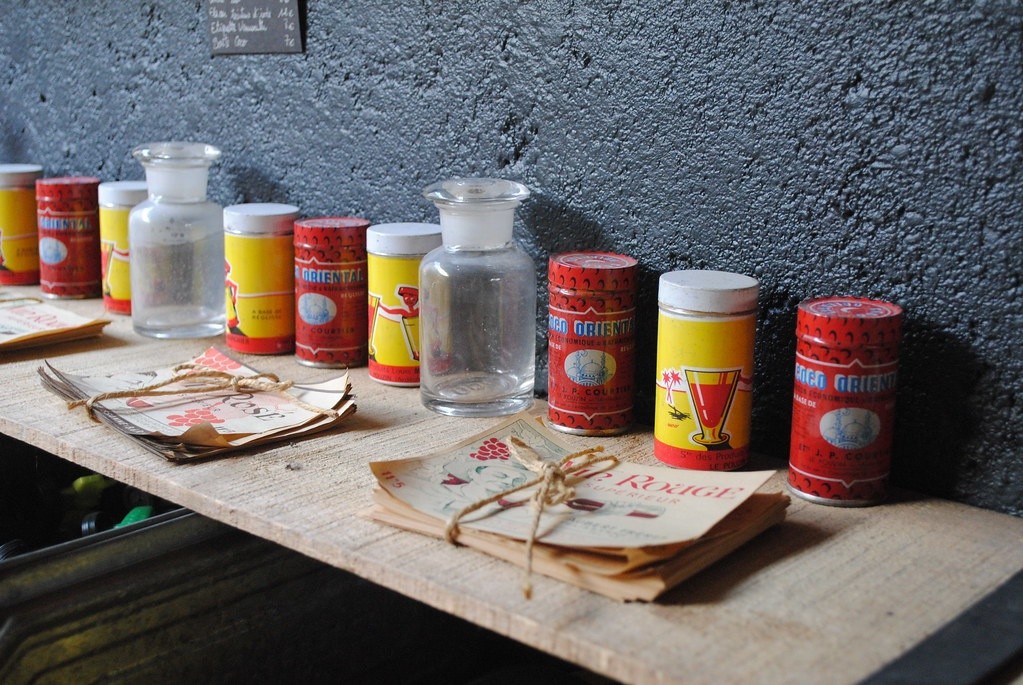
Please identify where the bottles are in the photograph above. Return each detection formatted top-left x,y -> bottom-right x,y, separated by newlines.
129,141 -> 223,340
417,179 -> 537,418
